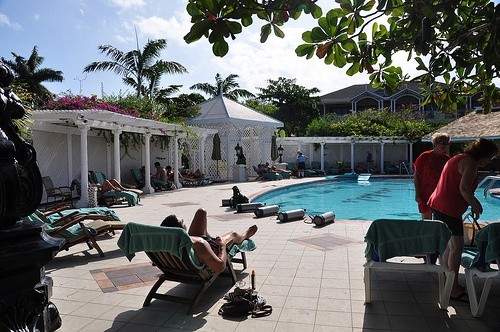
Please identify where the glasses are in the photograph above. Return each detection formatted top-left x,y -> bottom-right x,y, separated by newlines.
488,155 -> 495,160
441,141 -> 449,145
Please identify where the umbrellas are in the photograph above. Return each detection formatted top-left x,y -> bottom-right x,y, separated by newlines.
212,133 -> 222,176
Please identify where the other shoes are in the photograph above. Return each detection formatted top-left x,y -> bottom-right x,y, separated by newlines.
224,288 -> 257,302
450,293 -> 468,302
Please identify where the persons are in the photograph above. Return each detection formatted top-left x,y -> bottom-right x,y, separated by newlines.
160,207 -> 257,273
426,138 -> 499,304
88,179 -> 130,193
0,87 -> 43,228
414,133 -> 452,262
338,150 -> 415,175
257,162 -> 292,175
142,162 -> 204,192
296,150 -> 308,178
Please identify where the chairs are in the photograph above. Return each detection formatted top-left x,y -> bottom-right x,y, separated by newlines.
252,160 -> 420,182
364,219 -> 457,310
41,176 -> 73,205
458,221 -> 500,316
29,157 -> 213,257
127,222 -> 248,316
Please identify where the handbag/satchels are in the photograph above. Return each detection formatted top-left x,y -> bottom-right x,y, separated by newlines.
218,297 -> 272,315
